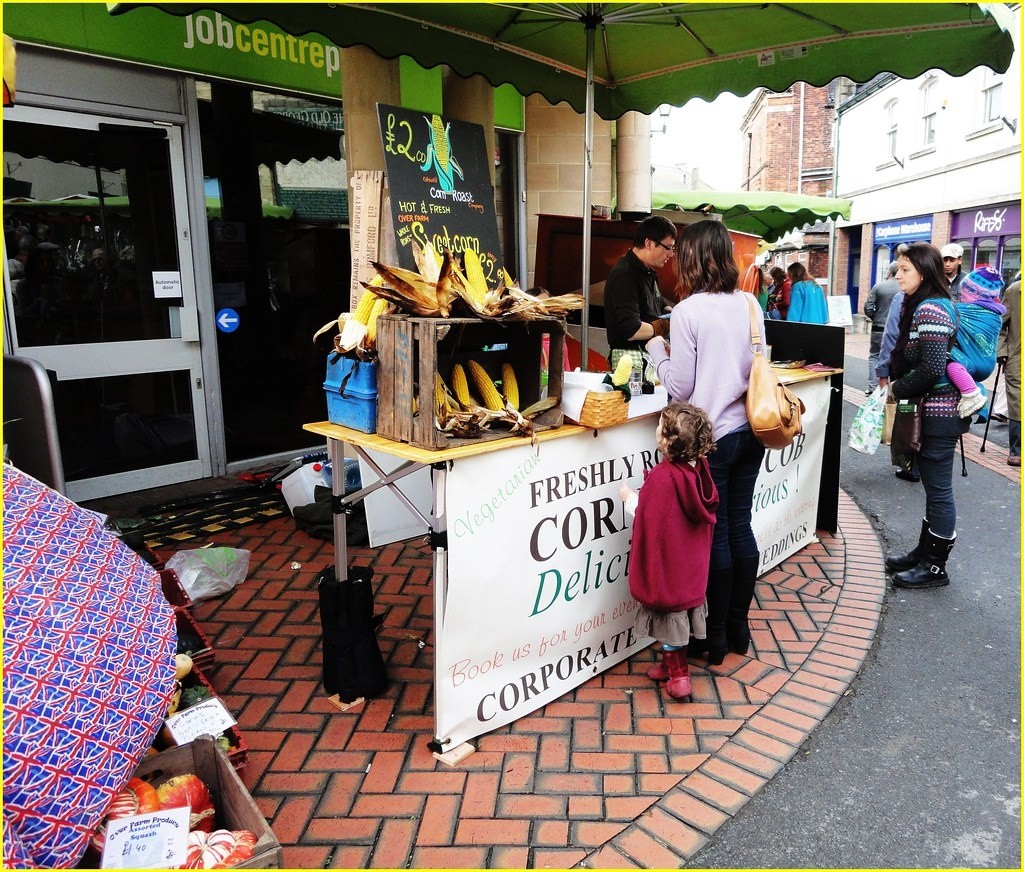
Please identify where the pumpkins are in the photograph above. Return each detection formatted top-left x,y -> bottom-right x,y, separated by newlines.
89,775 -> 259,871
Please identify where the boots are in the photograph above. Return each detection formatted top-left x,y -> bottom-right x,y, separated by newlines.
727,552 -> 760,654
687,560 -> 734,665
647,650 -> 668,680
886,516 -> 931,570
892,527 -> 957,587
664,647 -> 692,699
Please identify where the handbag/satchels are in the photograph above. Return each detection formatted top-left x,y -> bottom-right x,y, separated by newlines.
746,354 -> 806,449
764,301 -> 782,319
848,382 -> 922,467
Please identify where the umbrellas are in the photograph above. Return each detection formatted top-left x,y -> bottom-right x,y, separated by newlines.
106,3 -> 1014,371
611,191 -> 854,244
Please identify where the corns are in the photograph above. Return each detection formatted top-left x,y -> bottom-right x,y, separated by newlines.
435,359 -> 519,417
612,354 -> 633,386
354,274 -> 388,345
425,243 -> 487,306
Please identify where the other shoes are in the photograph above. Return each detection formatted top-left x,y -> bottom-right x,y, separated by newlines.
865,388 -> 871,394
990,412 -> 1007,422
1007,452 -> 1021,465
957,388 -> 987,418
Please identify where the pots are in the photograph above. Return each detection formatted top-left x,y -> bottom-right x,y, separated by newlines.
591,203 -> 612,218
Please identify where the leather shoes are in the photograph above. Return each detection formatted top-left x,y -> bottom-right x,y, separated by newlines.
896,468 -> 919,482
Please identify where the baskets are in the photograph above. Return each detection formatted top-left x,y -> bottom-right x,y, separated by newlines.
562,387 -> 629,428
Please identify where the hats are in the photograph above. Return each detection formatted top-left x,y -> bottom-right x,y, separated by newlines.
941,243 -> 964,259
960,266 -> 1005,302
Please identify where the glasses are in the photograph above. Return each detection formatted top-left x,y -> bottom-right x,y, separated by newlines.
650,238 -> 674,252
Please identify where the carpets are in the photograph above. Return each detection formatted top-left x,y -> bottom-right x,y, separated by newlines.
130,489 -> 289,548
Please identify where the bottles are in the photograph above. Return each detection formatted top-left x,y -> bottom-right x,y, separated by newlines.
322,459 -> 361,491
282,461 -> 329,516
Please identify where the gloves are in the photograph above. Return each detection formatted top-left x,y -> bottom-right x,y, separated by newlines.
997,357 -> 1008,363
645,336 -> 667,366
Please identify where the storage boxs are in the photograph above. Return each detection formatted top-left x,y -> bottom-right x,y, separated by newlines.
133,543 -> 285,869
322,316 -> 568,452
302,366 -> 849,768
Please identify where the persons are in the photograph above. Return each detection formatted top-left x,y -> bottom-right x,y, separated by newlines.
603,215 -> 678,394
786,262 -> 830,324
619,399 -> 719,698
947,266 -> 1007,419
884,241 -> 972,588
940,243 -> 968,299
996,280 -> 1021,466
875,291 -> 904,390
991,365 -> 1009,422
763,274 -> 781,319
645,221 -> 768,666
770,267 -> 792,320
758,268 -> 767,312
864,261 -> 901,396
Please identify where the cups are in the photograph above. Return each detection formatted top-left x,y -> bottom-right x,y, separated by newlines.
629,368 -> 643,396
